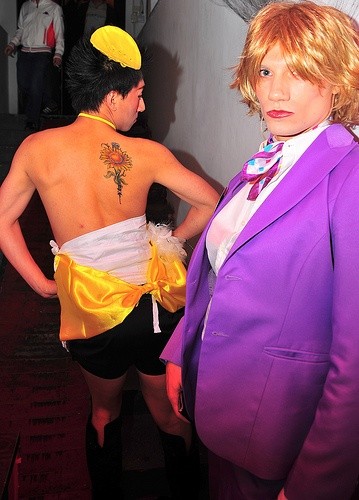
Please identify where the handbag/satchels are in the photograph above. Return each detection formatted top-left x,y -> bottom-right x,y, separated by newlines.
46,9 -> 57,48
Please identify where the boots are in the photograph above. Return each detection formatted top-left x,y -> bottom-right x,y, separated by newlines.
157,419 -> 203,500
85,413 -> 146,500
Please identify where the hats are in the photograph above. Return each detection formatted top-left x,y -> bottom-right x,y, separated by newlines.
90,25 -> 142,70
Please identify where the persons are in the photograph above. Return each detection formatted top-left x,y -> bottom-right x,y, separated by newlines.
160,0 -> 359,500
1,26 -> 221,500
4,0 -> 64,132
41,0 -> 119,114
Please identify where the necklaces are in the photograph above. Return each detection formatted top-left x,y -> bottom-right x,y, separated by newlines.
78,111 -> 117,129
